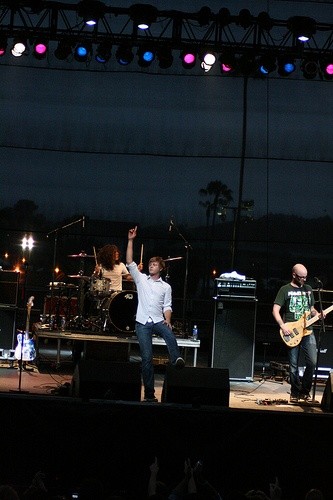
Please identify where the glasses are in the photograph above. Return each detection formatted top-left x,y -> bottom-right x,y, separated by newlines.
295,273 -> 307,280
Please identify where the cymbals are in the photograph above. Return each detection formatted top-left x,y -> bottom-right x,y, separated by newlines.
164,256 -> 182,261
68,253 -> 95,257
67,274 -> 92,278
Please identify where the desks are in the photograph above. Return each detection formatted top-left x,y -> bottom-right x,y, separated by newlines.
36,322 -> 200,371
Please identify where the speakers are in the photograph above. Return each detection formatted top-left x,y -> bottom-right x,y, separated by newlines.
70,361 -> 142,402
298,324 -> 333,370
0,270 -> 41,350
212,297 -> 258,382
161,366 -> 230,407
320,371 -> 333,413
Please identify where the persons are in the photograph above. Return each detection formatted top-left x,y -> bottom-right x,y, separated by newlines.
272,263 -> 326,402
95,244 -> 143,292
79,250 -> 87,256
126,226 -> 184,402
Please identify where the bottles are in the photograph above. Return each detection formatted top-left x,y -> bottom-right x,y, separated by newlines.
61,317 -> 66,332
192,325 -> 198,340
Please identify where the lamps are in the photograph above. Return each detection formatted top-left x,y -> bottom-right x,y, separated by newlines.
76,0 -> 105,25
198,6 -> 273,31
0,34 -> 333,81
130,4 -> 158,29
287,16 -> 316,40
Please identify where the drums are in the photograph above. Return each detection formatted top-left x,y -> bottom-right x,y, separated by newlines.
89,276 -> 111,299
83,291 -> 104,317
100,289 -> 139,334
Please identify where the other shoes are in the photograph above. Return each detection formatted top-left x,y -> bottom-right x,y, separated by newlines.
298,394 -> 313,403
174,356 -> 186,367
144,396 -> 158,402
290,393 -> 297,403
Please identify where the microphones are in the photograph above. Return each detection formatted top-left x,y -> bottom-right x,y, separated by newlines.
314,277 -> 323,286
169,221 -> 173,231
83,216 -> 85,228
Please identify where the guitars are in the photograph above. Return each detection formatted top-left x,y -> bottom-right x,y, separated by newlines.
14,296 -> 36,361
280,304 -> 333,347
